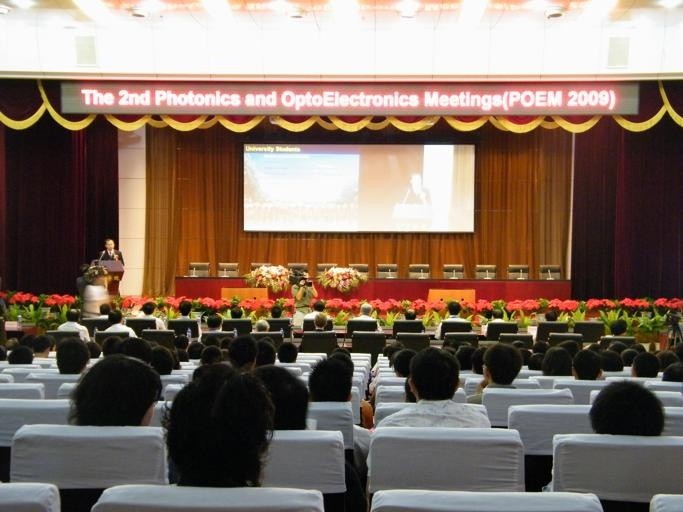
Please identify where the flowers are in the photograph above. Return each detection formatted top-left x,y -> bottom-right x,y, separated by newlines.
80,262 -> 109,285
316,266 -> 367,293
0,288 -> 81,329
242,265 -> 291,293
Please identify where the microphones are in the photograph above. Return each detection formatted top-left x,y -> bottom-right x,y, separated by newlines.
189,267 -> 199,277
325,268 -> 326,272
418,269 -> 427,280
450,268 -> 459,279
546,269 -> 555,281
517,269 -> 525,280
385,269 -> 395,278
484,269 -> 492,279
99,250 -> 105,260
220,268 -> 229,277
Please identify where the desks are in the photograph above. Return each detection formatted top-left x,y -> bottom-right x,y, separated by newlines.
173,276 -> 572,301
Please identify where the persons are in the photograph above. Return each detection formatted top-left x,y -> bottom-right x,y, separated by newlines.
1,298 -> 683,429
159,363 -> 277,487
100,239 -> 124,266
287,266 -> 320,329
400,173 -> 433,205
541,381 -> 664,492
